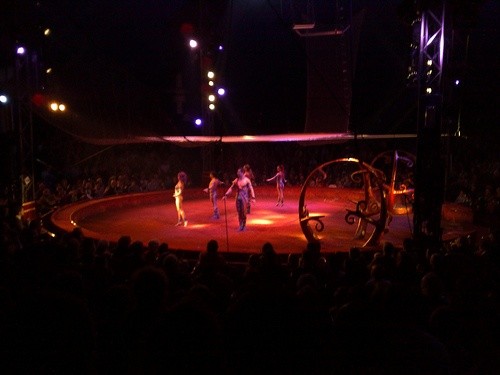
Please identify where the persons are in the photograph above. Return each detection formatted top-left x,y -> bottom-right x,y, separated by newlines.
267,165 -> 288,208
173,171 -> 191,227
222,165 -> 257,232
0,203 -> 500,375
218,157 -> 500,217
204,171 -> 220,219
34,163 -> 191,210
